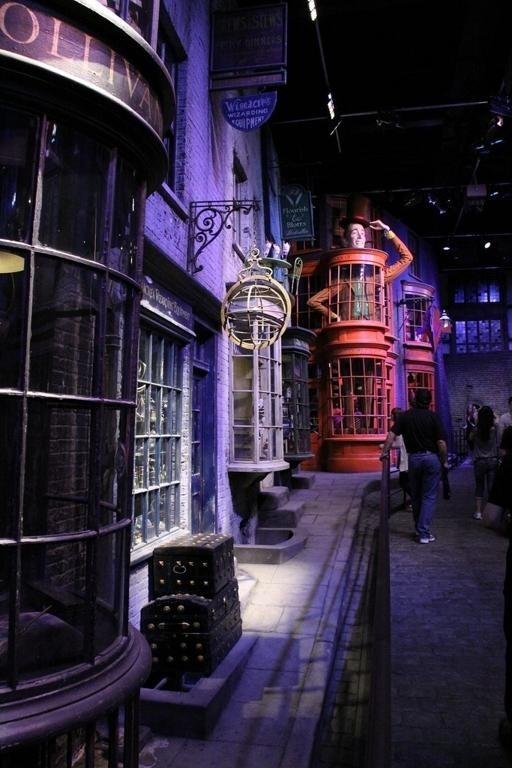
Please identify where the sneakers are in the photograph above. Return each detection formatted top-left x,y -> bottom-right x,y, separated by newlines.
419,534 -> 435,543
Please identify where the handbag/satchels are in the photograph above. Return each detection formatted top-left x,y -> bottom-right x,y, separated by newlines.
498,449 -> 506,466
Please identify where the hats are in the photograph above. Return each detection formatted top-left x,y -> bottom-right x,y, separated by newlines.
412,390 -> 432,408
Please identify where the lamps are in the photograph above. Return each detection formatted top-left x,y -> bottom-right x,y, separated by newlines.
189,195 -> 291,351
439,309 -> 450,328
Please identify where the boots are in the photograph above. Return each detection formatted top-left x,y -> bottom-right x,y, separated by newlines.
473,496 -> 483,520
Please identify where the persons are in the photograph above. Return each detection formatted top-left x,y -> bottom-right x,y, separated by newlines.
380,389 -> 450,544
307,211 -> 414,434
466,396 -> 512,520
481,425 -> 512,749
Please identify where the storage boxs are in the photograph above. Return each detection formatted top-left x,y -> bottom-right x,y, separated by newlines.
140,577 -> 242,678
145,530 -> 235,597
